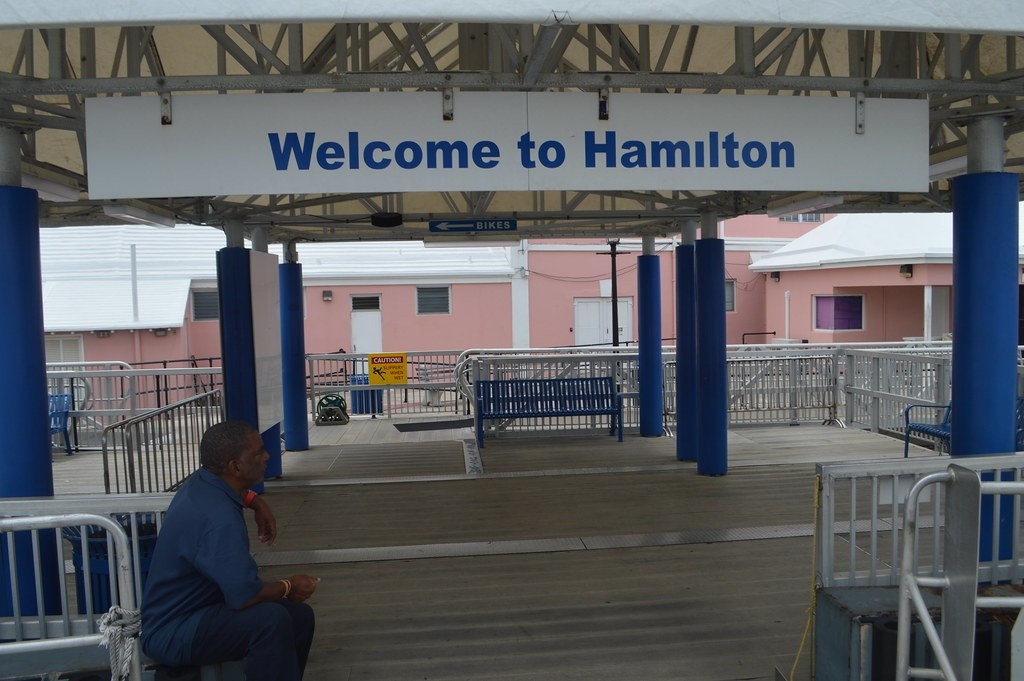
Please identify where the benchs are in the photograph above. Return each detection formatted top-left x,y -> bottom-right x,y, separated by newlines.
475,377 -> 623,448
903,395 -> 1024,458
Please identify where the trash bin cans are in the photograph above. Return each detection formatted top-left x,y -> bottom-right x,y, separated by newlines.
350,374 -> 383,414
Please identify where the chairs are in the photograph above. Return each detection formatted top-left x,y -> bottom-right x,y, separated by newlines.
49,393 -> 73,463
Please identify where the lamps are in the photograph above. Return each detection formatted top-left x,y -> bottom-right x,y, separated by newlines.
740,272 -> 780,289
97,331 -> 110,337
323,291 -> 332,300
899,265 -> 912,277
155,329 -> 167,336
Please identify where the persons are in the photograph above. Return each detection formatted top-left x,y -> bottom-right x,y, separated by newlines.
140,420 -> 320,681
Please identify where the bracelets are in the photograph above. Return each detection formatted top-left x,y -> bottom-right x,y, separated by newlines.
245,490 -> 256,506
278,578 -> 291,598
